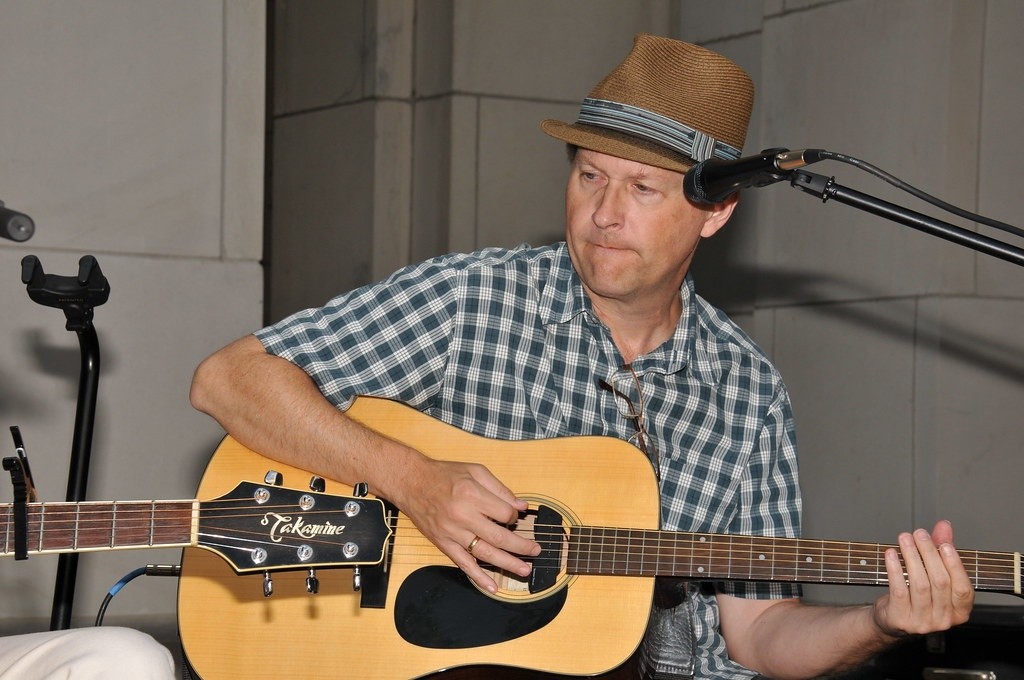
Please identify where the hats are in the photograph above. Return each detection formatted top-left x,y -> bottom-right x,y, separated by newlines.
540,32 -> 755,174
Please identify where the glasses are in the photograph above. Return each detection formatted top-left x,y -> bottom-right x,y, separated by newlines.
597,363 -> 661,483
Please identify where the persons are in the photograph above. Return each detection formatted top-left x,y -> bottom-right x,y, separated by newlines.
0,606 -> 178,677
191,35 -> 974,679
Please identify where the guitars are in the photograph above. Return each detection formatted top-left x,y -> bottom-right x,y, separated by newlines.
178,396 -> 1024,680
0,469 -> 391,596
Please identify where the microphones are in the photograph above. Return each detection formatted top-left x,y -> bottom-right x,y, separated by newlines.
0,199 -> 35,243
683,147 -> 829,205
